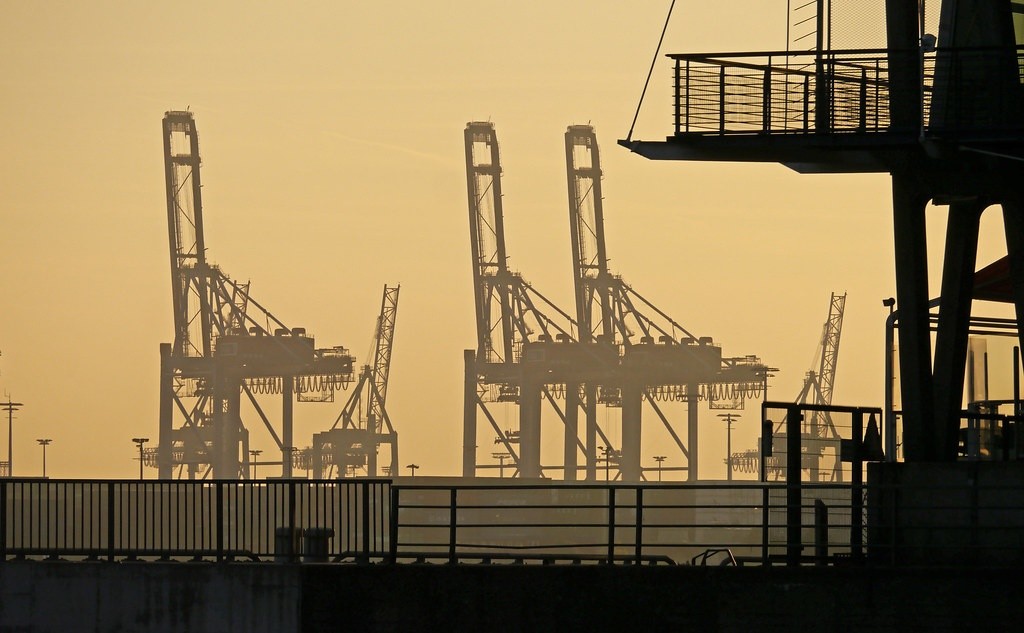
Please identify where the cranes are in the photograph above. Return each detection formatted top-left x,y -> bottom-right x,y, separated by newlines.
159,103 -> 399,482
461,118 -> 849,484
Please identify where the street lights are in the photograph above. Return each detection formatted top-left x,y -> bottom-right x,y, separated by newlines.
407,464 -> 419,476
597,447 -> 612,482
0,403 -> 24,478
491,453 -> 511,481
716,413 -> 743,480
653,456 -> 668,482
37,439 -> 53,478
247,450 -> 264,480
130,437 -> 150,480
